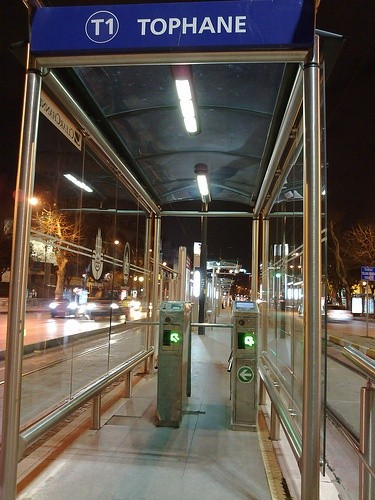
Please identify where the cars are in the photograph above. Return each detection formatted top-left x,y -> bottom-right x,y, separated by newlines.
48,297 -> 132,320
324,307 -> 355,324
134,295 -> 153,312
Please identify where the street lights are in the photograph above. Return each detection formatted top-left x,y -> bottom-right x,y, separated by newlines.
363,280 -> 368,338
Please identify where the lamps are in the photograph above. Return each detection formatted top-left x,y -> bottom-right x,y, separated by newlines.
194,163 -> 212,209
172,60 -> 202,137
63,171 -> 93,194
